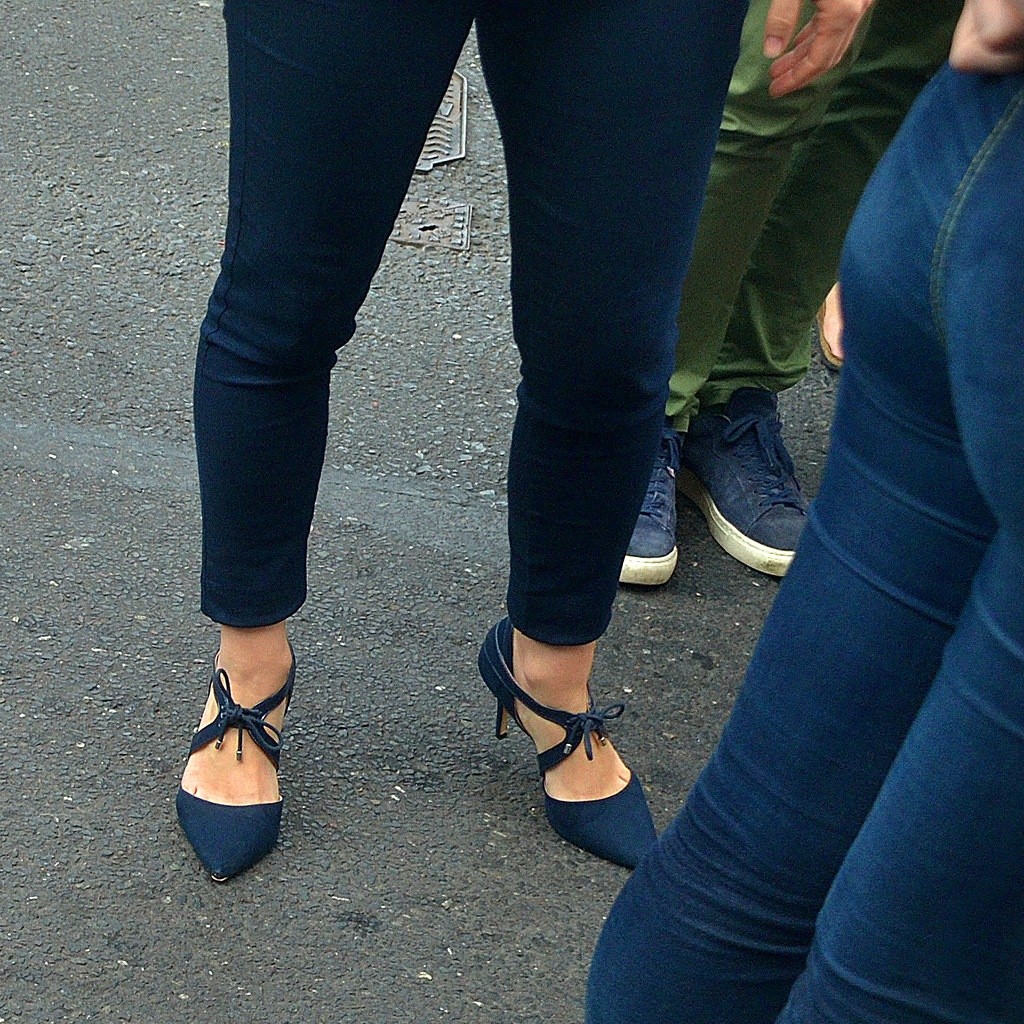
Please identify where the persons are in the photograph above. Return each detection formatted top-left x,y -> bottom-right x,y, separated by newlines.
584,0 -> 1024,1024
174,0 -> 752,881
620,0 -> 963,587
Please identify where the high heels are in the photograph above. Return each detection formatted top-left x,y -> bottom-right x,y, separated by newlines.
477,616 -> 656,869
176,636 -> 295,882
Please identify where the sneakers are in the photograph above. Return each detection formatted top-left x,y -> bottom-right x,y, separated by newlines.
674,388 -> 809,578
617,414 -> 682,586
